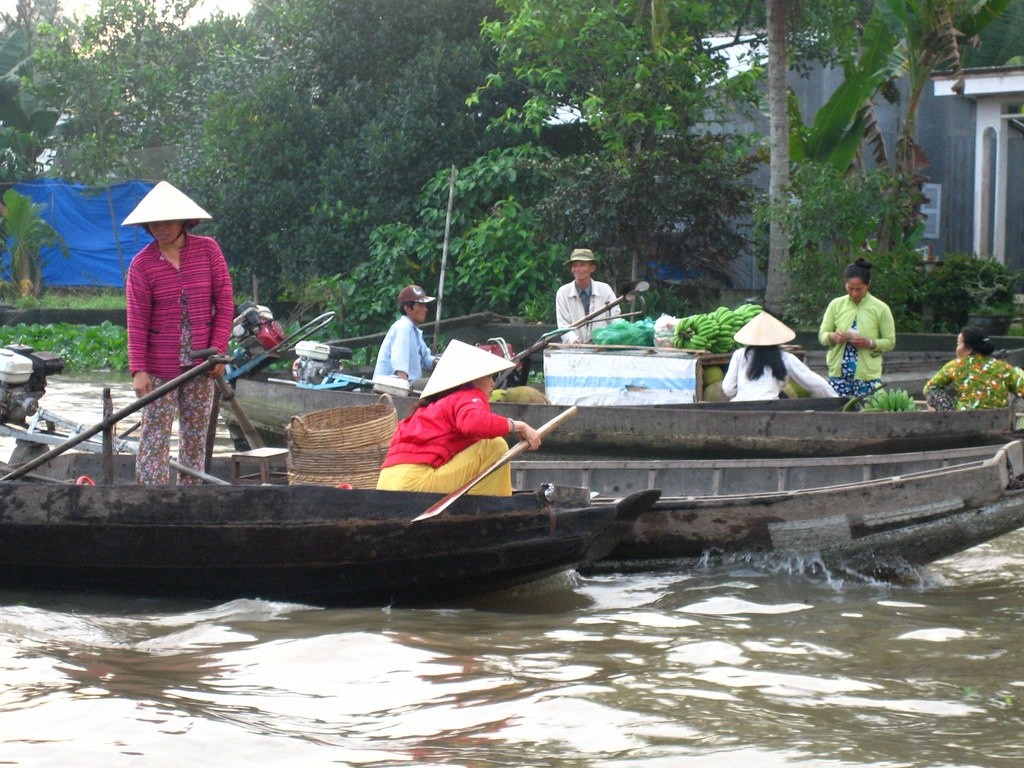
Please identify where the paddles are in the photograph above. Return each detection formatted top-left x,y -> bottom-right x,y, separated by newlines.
413,403 -> 581,524
295,372 -> 411,398
0,351 -> 218,482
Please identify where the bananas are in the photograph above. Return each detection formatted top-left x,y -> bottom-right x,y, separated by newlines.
672,303 -> 767,353
862,387 -> 916,413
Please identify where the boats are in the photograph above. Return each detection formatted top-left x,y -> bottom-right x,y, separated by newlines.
0,345 -> 1024,582
0,479 -> 664,607
227,345 -> 1024,462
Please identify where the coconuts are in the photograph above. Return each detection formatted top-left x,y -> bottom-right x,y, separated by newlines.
701,365 -> 729,401
779,379 -> 810,398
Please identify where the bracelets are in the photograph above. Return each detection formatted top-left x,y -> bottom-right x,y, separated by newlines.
869,340 -> 873,347
508,418 -> 515,435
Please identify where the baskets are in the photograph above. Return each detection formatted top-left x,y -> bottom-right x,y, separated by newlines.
284,393 -> 398,489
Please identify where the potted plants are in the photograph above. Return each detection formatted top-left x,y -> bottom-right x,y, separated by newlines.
969,302 -> 1013,337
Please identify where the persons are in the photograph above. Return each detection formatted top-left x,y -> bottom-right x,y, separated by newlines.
556,248 -> 621,348
121,181 -> 234,485
377,340 -> 542,496
922,325 -> 1024,411
722,312 -> 838,401
818,259 -> 897,400
372,285 -> 439,398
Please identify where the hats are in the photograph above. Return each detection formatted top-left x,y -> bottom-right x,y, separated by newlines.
120,180 -> 213,226
562,249 -> 600,275
399,285 -> 437,303
420,337 -> 516,398
734,311 -> 797,346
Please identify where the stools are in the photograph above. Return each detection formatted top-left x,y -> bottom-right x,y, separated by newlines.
231,447 -> 289,487
